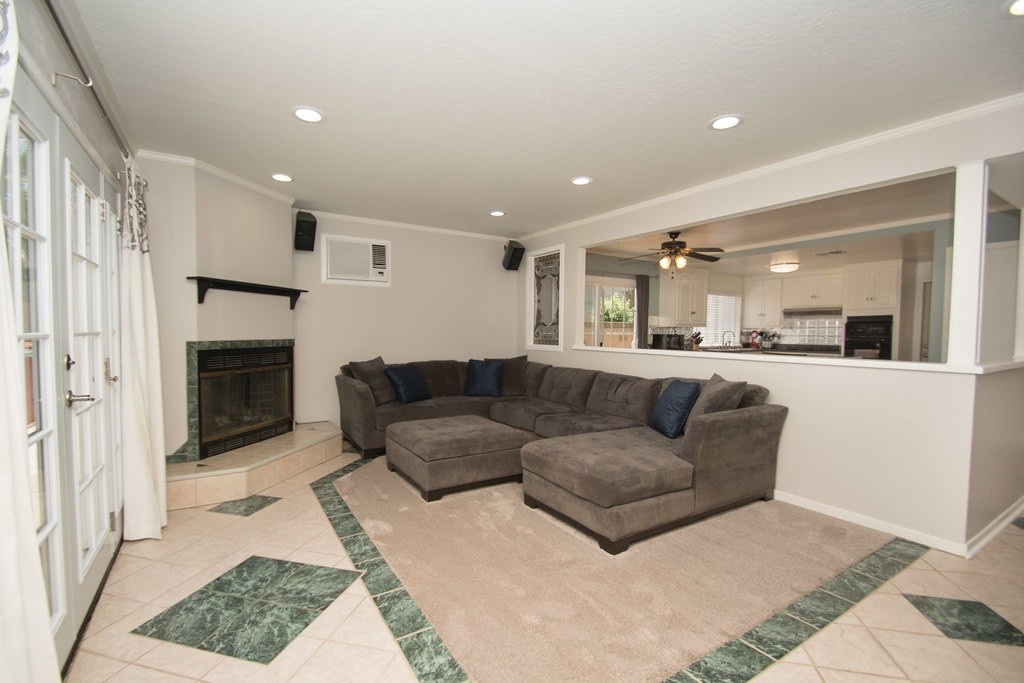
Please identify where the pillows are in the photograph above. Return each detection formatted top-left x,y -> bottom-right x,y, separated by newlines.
463,359 -> 505,397
681,373 -> 747,437
383,364 -> 432,404
648,380 -> 700,439
349,356 -> 398,408
484,355 -> 530,396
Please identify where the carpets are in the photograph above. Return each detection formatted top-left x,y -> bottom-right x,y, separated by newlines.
331,450 -> 897,683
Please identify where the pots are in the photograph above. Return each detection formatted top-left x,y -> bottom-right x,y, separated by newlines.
762,340 -> 773,348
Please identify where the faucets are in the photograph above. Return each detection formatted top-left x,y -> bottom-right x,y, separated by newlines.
722,330 -> 736,349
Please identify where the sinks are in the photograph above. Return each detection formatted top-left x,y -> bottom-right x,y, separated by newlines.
703,347 -> 752,352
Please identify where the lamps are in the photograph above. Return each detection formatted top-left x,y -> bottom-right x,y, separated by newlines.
658,251 -> 688,270
769,263 -> 800,273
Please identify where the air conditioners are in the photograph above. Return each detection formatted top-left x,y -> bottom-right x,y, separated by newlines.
320,232 -> 392,288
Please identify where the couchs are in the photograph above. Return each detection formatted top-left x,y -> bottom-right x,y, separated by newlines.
335,354 -> 789,556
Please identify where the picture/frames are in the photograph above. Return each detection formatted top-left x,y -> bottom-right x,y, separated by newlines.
525,243 -> 564,353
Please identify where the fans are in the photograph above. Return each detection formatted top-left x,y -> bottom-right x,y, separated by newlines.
618,230 -> 725,263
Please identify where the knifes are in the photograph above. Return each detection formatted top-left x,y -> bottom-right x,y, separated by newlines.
691,331 -> 703,344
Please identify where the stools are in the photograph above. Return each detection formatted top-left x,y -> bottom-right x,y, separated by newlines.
385,414 -> 532,502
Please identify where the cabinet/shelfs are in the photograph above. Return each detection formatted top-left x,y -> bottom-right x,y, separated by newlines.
741,257 -> 917,361
659,264 -> 711,328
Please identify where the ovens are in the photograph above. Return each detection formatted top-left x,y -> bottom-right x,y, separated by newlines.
845,316 -> 892,360
